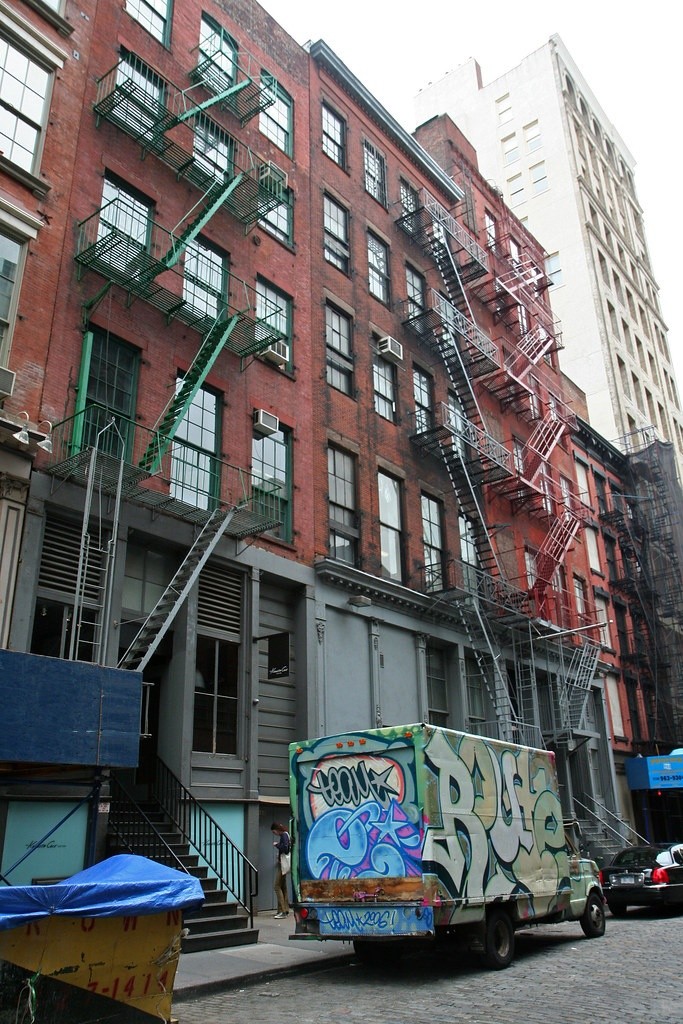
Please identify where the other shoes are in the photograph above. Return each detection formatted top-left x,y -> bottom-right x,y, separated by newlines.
274,911 -> 289,918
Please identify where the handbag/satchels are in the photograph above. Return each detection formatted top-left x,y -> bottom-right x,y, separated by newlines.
280,832 -> 291,876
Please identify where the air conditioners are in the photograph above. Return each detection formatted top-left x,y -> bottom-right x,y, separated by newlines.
254,409 -> 279,434
259,160 -> 288,192
378,335 -> 404,361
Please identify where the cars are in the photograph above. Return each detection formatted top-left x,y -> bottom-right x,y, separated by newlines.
596,841 -> 683,918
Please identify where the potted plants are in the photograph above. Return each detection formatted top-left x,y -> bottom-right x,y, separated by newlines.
261,335 -> 290,365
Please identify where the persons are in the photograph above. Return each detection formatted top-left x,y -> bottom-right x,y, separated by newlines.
270,822 -> 292,919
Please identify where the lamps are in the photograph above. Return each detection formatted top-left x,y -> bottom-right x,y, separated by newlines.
37,420 -> 53,454
347,594 -> 371,609
11,410 -> 30,445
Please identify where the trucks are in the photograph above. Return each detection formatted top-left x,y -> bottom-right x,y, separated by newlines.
288,719 -> 606,974
